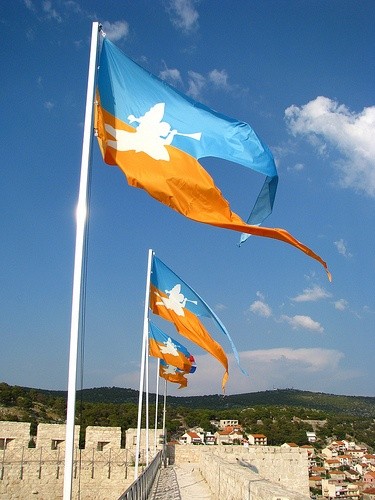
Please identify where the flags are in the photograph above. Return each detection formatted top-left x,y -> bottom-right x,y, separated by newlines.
148,319 -> 196,374
97,34 -> 335,286
149,255 -> 249,377
159,357 -> 188,390
